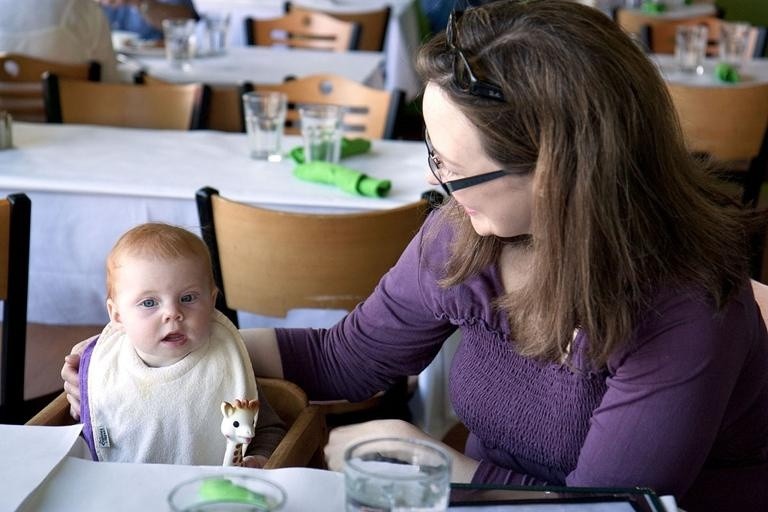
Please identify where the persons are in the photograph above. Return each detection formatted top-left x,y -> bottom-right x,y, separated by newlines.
63,0 -> 768,511
96,1 -> 165,41
78,221 -> 288,472
1,0 -> 124,83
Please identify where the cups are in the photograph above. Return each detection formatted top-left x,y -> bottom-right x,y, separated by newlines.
298,104 -> 347,165
670,23 -> 709,77
718,22 -> 761,74
241,90 -> 289,160
341,436 -> 451,511
160,9 -> 232,72
0,113 -> 13,150
168,474 -> 288,511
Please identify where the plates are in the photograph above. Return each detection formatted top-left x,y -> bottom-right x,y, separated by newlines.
114,36 -> 226,60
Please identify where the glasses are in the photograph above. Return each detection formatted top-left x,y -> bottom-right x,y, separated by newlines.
420,125 -> 538,197
435,7 -> 520,108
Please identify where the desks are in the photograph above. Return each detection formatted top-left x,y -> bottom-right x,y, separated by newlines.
0,0 -> 768,512
